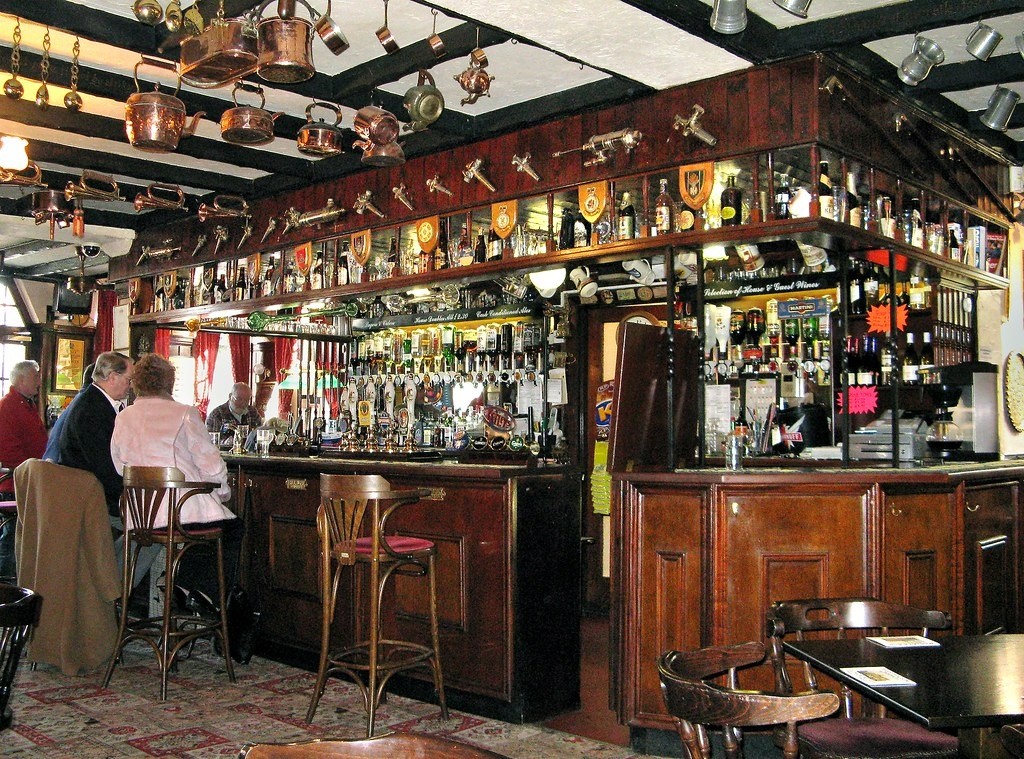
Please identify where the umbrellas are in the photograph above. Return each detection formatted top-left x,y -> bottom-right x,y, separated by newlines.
277,371 -> 344,389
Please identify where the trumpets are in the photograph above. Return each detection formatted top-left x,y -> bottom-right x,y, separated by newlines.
199,195 -> 252,223
64,171 -> 126,202
134,183 -> 188,213
0,160 -> 49,189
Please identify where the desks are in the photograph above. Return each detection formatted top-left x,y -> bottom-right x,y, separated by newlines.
782,634 -> 1024,759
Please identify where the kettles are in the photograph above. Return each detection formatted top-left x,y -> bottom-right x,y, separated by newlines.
125,0 -> 494,166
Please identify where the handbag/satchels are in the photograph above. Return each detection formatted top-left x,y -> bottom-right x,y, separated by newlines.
212,586 -> 261,665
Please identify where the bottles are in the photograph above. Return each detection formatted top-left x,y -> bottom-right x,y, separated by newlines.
849,259 -> 890,314
721,175 -> 742,227
706,418 -> 721,454
819,316 -> 830,357
766,299 -> 782,357
735,406 -> 748,455
846,332 -> 933,387
785,318 -> 799,357
339,317 -> 559,453
774,174 -> 791,220
155,178 -> 695,312
846,171 -> 862,229
704,304 -> 764,360
725,417 -> 742,471
802,316 -> 817,358
950,229 -> 960,261
896,270 -> 932,309
818,160 -> 833,220
911,198 -> 922,248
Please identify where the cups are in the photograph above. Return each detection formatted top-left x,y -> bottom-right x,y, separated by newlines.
833,186 -> 843,223
569,240 -> 827,298
227,317 -> 337,335
208,425 -> 274,457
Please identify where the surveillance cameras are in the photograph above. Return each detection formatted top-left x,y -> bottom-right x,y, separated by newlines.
82,242 -> 101,258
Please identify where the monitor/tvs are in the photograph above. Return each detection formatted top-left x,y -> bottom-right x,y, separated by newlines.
57,282 -> 93,314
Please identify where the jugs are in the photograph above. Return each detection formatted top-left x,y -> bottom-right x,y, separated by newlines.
926,411 -> 963,450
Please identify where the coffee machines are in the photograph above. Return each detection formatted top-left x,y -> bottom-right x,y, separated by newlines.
915,361 -> 1000,462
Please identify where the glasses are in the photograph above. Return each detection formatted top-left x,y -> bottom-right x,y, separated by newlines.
118,373 -> 134,385
231,395 -> 251,405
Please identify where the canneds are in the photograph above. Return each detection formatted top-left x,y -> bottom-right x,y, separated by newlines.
328,420 -> 337,434
846,334 -> 878,353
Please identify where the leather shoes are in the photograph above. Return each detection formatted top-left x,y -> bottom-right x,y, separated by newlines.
186,590 -> 218,622
171,586 -> 194,615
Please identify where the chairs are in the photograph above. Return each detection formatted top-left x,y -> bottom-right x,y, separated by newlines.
0,582 -> 40,732
239,734 -> 510,759
774,597 -> 959,759
657,640 -> 840,759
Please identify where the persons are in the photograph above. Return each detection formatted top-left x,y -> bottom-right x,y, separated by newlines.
40,352 -> 163,594
109,353 -> 245,614
205,380 -> 264,445
0,359 -> 49,587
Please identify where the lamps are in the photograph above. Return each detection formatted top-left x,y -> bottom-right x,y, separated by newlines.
0,135 -> 29,173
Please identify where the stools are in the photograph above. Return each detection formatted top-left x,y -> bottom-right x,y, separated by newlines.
0,468 -> 17,530
99,467 -> 238,701
13,458 -> 124,675
304,472 -> 450,740
